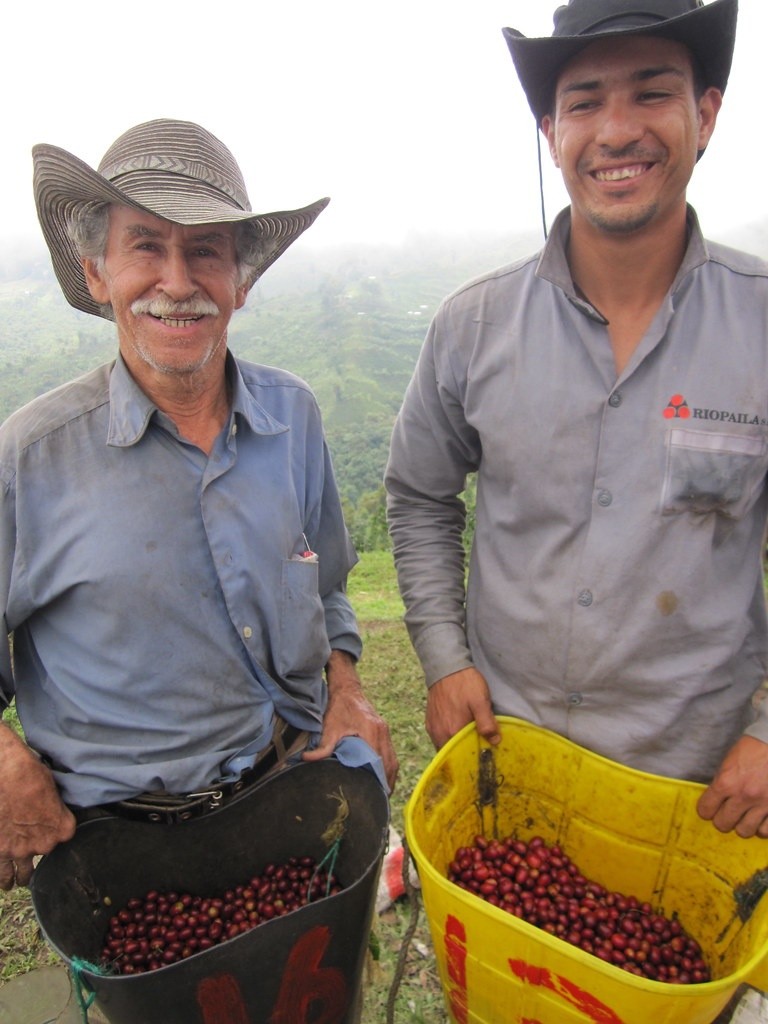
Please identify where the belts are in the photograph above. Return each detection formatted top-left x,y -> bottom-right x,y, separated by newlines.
102,720 -> 302,828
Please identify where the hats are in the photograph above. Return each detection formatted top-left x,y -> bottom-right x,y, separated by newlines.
502,0 -> 738,126
33,118 -> 330,324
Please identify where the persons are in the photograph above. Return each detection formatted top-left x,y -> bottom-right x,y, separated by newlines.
0,117 -> 400,895
381,1 -> 767,1024
